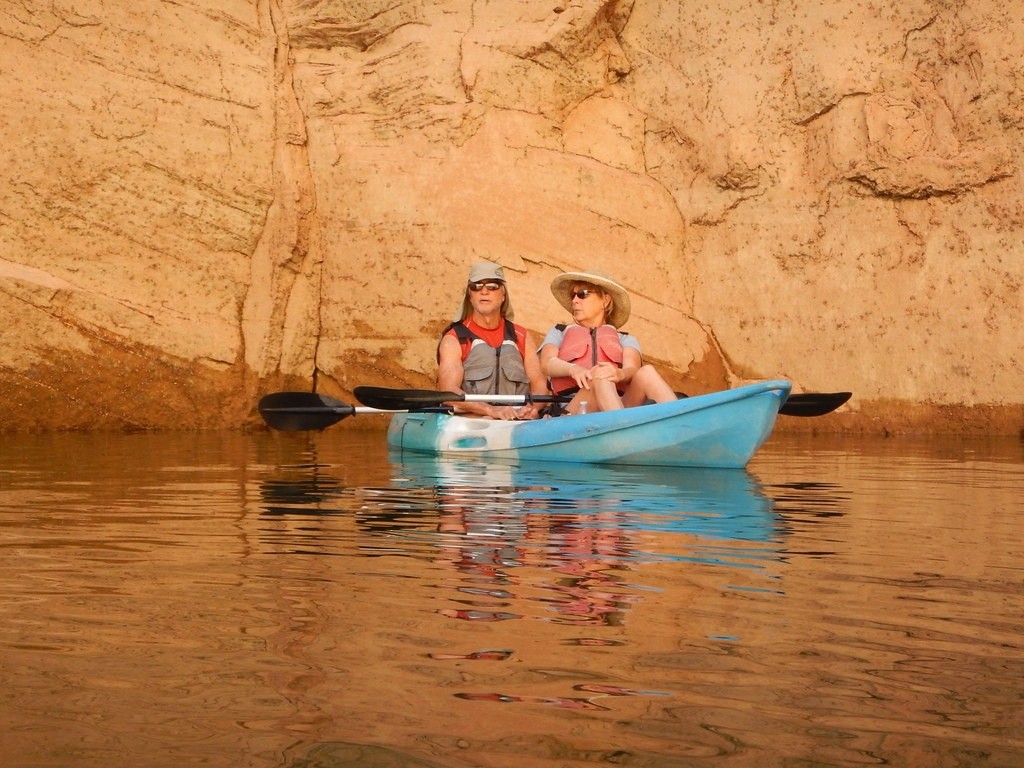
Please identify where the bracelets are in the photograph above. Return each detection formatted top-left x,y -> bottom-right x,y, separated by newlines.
569,364 -> 576,378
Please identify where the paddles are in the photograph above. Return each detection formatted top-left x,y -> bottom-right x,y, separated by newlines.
352,383 -> 854,418
256,388 -> 692,432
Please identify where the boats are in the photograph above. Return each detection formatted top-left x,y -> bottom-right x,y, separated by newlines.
391,447 -> 790,548
386,378 -> 793,469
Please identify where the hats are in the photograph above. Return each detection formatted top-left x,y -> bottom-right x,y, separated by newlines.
450,261 -> 516,324
550,273 -> 632,328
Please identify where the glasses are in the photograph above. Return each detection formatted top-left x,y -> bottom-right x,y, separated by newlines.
570,290 -> 609,300
470,282 -> 502,292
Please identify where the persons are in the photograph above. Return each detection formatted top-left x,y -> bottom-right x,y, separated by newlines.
437,261 -> 549,420
537,272 -> 678,416
